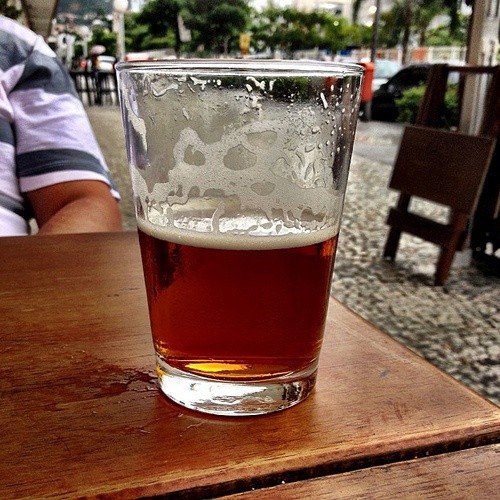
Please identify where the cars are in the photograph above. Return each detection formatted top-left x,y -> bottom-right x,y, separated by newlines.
372,63 -> 465,125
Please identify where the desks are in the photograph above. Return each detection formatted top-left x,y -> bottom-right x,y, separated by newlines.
1,231 -> 499,500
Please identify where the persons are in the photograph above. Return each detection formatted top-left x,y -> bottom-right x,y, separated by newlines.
0,15 -> 125,238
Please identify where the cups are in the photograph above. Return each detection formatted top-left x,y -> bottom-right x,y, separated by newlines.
115,59 -> 365,416
